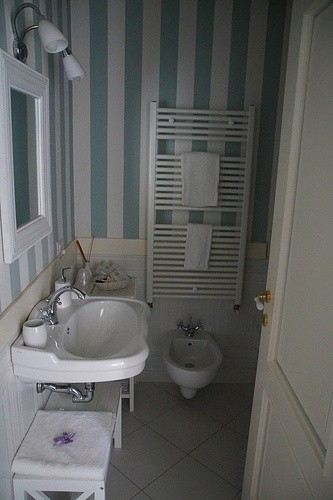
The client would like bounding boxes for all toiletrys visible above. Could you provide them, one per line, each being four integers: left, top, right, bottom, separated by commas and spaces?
54, 266, 73, 310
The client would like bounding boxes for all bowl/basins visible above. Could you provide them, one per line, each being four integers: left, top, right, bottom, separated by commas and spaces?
93, 275, 129, 290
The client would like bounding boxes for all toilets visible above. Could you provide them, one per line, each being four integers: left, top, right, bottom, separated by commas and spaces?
167, 315, 223, 401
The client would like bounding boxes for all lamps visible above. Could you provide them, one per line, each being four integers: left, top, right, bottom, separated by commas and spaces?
12, 2, 86, 82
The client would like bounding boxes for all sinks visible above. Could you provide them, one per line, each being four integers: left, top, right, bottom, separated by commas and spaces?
55, 297, 145, 369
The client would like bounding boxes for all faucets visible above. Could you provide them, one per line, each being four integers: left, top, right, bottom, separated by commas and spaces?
37, 284, 88, 327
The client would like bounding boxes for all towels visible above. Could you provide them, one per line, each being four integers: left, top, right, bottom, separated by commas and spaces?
11, 405, 117, 480
184, 224, 211, 272
181, 152, 220, 209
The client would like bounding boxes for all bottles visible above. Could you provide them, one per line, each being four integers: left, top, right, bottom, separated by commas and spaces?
75, 268, 94, 295
55, 267, 71, 308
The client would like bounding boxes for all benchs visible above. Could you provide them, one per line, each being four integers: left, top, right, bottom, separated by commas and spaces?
13, 382, 122, 500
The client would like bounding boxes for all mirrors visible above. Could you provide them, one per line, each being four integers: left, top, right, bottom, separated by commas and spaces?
0, 48, 53, 264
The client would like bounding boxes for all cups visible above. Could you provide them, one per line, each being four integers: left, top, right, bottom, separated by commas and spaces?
23, 319, 47, 349
